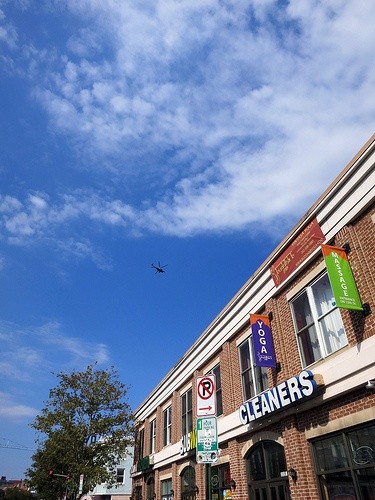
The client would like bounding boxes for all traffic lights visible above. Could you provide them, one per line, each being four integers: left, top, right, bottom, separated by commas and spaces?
48, 469, 53, 477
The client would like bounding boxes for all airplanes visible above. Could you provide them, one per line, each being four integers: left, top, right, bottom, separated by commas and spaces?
151, 260, 169, 275
